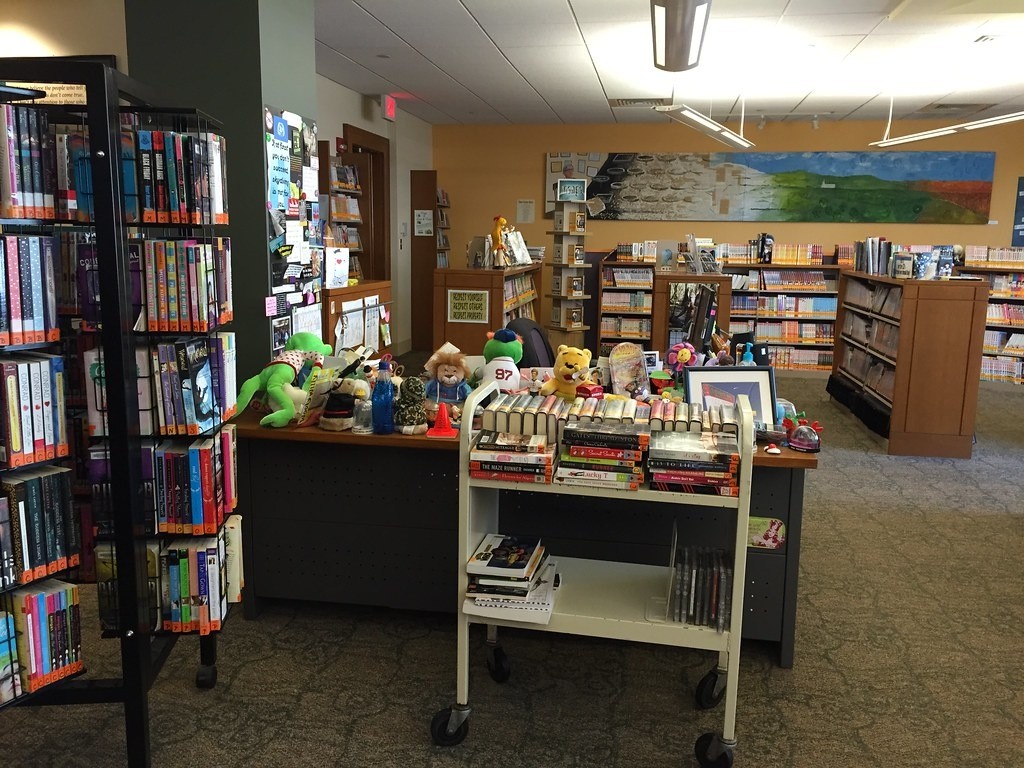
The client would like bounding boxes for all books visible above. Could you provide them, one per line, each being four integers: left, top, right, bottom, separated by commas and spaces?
470, 392, 740, 498
961, 245, 1024, 384
0, 103, 244, 703
718, 245, 855, 370
600, 240, 656, 359
466, 534, 556, 611
890, 244, 932, 257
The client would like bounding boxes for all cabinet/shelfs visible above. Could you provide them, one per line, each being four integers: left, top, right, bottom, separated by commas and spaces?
0, 54, 1024, 768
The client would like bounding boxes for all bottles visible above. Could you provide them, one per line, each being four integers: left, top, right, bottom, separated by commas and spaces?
364, 365, 376, 389
372, 363, 395, 435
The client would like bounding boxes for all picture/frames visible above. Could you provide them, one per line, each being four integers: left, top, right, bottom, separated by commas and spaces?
683, 365, 778, 430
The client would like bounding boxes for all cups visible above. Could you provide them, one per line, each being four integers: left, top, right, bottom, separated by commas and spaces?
351, 400, 372, 435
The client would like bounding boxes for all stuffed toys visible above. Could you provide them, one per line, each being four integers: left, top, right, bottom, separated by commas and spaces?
395, 329, 524, 436
229, 331, 332, 428
662, 343, 698, 403
542, 345, 592, 402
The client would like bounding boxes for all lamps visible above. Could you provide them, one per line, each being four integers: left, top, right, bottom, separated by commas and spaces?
811, 114, 820, 130
867, 94, 1024, 148
643, 80, 756, 150
649, 0, 714, 73
757, 115, 767, 130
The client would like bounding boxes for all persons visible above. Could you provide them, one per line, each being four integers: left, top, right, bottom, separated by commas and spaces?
528, 369, 543, 397
0, 660, 22, 703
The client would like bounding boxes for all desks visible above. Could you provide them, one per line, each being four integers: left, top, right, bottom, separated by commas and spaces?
232, 408, 822, 669
